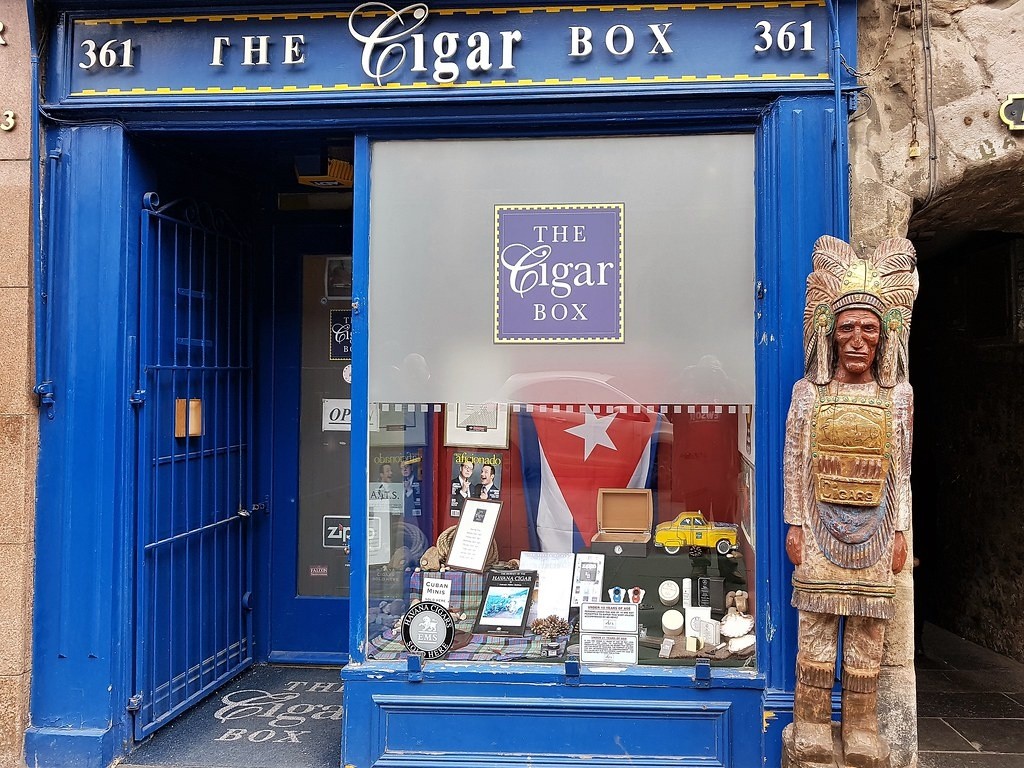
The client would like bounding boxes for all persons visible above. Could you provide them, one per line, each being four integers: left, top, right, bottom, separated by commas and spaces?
784, 235, 917, 765
374, 457, 419, 511
673, 356, 745, 583
450, 459, 499, 511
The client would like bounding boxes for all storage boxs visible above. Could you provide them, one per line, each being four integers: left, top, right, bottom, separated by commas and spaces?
590, 487, 654, 558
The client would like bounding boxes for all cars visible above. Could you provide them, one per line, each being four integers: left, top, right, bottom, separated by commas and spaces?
461, 371, 675, 490
653, 510, 739, 556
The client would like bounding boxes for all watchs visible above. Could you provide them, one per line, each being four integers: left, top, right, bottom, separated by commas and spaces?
633, 587, 640, 603
613, 586, 621, 602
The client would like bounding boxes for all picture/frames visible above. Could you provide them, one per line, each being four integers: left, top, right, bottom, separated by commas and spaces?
444, 498, 504, 573
443, 403, 510, 449
